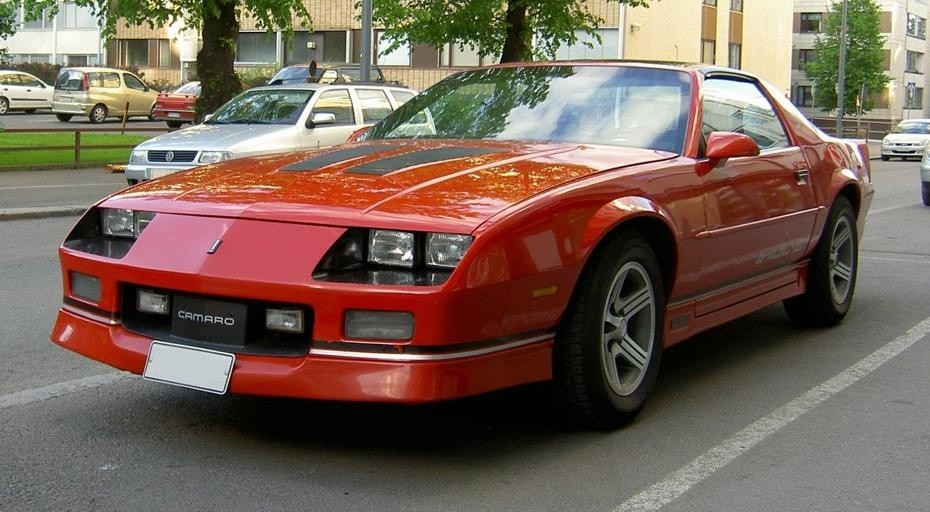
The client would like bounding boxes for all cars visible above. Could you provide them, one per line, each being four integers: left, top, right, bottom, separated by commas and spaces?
0, 70, 55, 116
879, 117, 930, 163
919, 140, 930, 207
44, 54, 873, 434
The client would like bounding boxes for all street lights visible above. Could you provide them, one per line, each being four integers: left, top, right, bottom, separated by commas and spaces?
905, 82, 917, 119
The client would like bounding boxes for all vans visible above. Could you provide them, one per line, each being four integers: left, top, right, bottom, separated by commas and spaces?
124, 81, 440, 189
